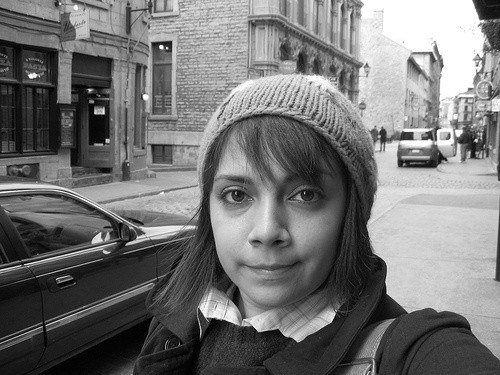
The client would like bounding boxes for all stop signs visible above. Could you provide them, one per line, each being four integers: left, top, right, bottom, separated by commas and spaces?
359, 102, 366, 110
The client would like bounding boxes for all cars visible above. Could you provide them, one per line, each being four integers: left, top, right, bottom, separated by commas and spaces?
0, 175, 197, 375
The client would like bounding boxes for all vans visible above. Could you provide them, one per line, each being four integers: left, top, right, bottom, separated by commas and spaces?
397, 127, 463, 168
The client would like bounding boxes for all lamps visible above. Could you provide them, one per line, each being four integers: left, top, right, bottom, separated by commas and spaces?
54, 1, 78, 10
352, 63, 370, 77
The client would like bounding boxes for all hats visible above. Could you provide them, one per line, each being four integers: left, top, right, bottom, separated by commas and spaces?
196, 74, 377, 226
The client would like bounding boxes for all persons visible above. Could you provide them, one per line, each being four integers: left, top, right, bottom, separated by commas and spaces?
458, 124, 489, 163
131, 74, 500, 375
371, 126, 378, 144
378, 126, 386, 152
